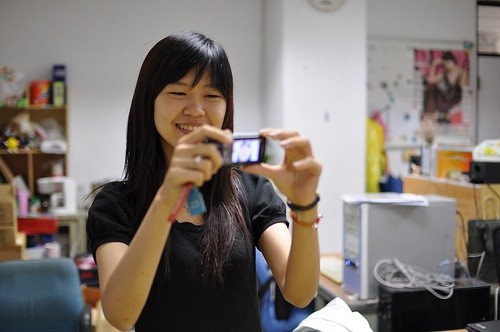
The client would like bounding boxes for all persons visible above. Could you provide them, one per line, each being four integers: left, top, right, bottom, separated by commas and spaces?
85, 31, 321, 332
235, 142, 251, 162
420, 51, 468, 120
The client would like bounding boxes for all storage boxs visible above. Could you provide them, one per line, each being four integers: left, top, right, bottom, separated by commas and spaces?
0, 184, 26, 261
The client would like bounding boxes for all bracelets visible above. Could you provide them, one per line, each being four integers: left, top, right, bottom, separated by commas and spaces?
289, 210, 323, 229
287, 194, 320, 211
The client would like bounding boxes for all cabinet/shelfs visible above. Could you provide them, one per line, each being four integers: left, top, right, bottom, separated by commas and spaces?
0, 103, 69, 199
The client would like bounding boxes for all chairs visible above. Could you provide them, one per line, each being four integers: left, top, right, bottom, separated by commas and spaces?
0, 258, 91, 332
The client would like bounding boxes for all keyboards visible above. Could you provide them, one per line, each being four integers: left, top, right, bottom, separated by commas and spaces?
320, 256, 344, 284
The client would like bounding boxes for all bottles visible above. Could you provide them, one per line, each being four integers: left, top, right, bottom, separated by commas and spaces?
51, 65, 68, 105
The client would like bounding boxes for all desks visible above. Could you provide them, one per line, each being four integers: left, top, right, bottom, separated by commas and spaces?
403, 174, 500, 268
314, 250, 380, 313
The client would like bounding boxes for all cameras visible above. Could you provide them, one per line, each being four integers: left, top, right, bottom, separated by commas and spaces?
222, 134, 267, 167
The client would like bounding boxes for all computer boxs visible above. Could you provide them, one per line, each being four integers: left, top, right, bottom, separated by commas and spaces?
342, 194, 456, 301
377, 278, 491, 332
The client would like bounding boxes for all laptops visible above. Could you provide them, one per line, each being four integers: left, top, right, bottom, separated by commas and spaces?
466, 321, 500, 332
